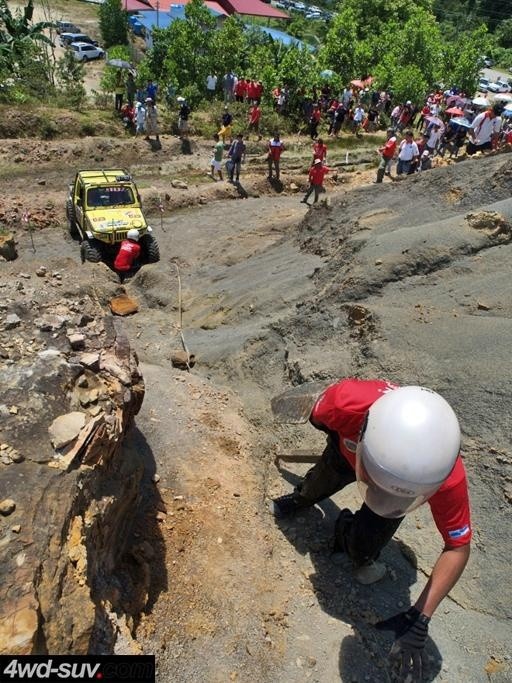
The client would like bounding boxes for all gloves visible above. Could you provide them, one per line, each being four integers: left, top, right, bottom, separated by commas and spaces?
373, 606, 436, 683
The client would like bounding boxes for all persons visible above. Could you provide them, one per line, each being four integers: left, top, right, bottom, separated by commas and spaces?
113, 229, 141, 284
267, 378, 472, 683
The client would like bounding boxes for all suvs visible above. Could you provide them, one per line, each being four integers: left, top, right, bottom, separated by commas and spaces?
67, 167, 159, 265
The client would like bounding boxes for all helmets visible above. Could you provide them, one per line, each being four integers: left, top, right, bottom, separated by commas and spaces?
355, 381, 461, 519
125, 228, 140, 242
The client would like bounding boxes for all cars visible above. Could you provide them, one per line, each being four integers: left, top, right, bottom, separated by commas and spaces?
476, 76, 490, 92
276, 0, 342, 25
57, 19, 106, 63
475, 54, 492, 68
499, 75, 512, 88
489, 80, 511, 94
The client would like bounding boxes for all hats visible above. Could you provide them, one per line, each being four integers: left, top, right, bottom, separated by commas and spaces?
176, 95, 186, 103
311, 157, 325, 167
135, 96, 154, 109
493, 104, 504, 116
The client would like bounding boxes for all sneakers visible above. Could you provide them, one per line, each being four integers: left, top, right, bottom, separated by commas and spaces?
297, 198, 309, 204
330, 509, 356, 567
205, 172, 240, 185
264, 175, 281, 180
266, 486, 315, 520
